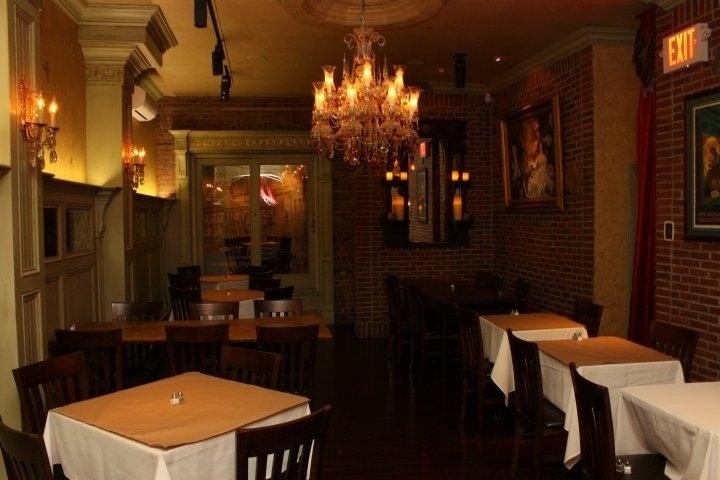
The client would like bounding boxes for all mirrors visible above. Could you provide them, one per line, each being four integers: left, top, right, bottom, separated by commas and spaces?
397, 119, 471, 246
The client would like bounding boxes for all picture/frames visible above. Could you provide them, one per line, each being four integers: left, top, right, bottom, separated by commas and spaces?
500, 92, 565, 213
417, 167, 428, 223
682, 87, 720, 240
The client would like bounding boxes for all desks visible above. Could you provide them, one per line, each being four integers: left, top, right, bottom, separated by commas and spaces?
615, 380, 720, 480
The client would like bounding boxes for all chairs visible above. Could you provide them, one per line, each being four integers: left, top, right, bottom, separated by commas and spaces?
480, 269, 533, 308
220, 234, 293, 274
379, 271, 456, 376
454, 305, 504, 436
574, 296, 604, 336
505, 328, 565, 479
568, 361, 651, 480
650, 319, 699, 379
0, 263, 338, 480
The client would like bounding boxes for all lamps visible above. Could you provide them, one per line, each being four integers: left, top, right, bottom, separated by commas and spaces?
449, 171, 471, 221
192, 0, 235, 101
386, 171, 408, 221
123, 142, 148, 186
19, 90, 60, 167
661, 22, 711, 75
306, 0, 423, 167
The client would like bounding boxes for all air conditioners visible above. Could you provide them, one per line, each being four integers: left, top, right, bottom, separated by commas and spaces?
132, 85, 159, 123
